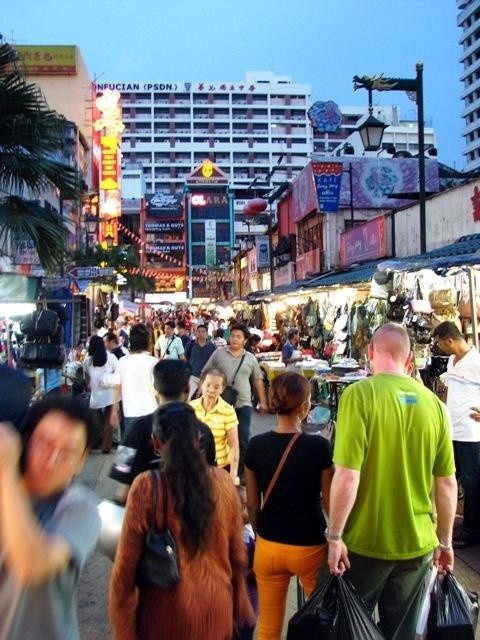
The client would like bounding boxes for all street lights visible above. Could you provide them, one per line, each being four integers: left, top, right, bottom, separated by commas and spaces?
243, 213, 274, 291
82, 210, 98, 261
105, 234, 114, 258
352, 62, 427, 257
222, 244, 244, 298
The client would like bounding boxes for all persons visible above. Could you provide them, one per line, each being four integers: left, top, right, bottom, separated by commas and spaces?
1, 302, 480, 640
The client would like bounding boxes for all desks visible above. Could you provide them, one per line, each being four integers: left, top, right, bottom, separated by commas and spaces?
252, 346, 369, 422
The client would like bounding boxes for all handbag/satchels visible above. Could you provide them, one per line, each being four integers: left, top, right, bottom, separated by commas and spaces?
132, 522, 183, 594
100, 369, 123, 390
219, 384, 239, 408
